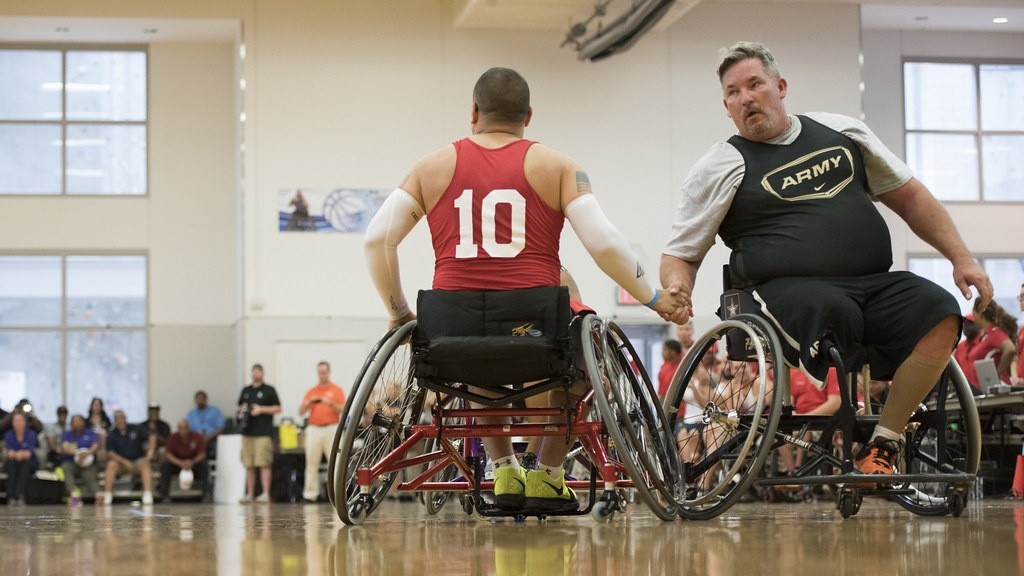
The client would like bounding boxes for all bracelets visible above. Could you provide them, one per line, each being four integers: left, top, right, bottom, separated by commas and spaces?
644, 291, 659, 307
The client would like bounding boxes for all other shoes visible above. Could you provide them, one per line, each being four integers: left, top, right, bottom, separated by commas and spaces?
69, 498, 85, 507
104, 492, 113, 504
239, 495, 255, 503
256, 493, 271, 502
141, 490, 154, 503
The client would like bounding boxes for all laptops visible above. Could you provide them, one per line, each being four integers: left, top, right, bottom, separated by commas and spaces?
974, 358, 1001, 394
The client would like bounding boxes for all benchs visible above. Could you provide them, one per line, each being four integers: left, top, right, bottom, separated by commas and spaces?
0, 460, 215, 502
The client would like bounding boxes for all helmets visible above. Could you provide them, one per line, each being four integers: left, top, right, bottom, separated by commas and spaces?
148, 403, 160, 411
73, 447, 94, 468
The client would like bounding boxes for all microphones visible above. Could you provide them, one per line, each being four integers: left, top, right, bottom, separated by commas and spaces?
989, 384, 1024, 393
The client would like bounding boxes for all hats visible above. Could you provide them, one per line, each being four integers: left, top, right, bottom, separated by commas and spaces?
963, 314, 974, 323
179, 468, 194, 490
666, 341, 682, 354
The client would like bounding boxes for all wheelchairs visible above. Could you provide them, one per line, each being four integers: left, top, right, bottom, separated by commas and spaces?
327, 285, 685, 526
662, 264, 983, 520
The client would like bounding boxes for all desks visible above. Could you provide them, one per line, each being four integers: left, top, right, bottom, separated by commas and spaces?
921, 389, 1024, 490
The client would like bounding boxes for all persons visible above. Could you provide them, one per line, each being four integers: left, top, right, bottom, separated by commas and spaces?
366, 67, 693, 508
0, 286, 1024, 505
658, 42, 992, 490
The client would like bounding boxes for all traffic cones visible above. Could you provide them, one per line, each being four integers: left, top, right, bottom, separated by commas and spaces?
1012, 455, 1024, 497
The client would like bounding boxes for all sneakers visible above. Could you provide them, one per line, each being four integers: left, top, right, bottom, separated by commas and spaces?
852, 435, 900, 487
523, 469, 579, 508
493, 467, 527, 508
